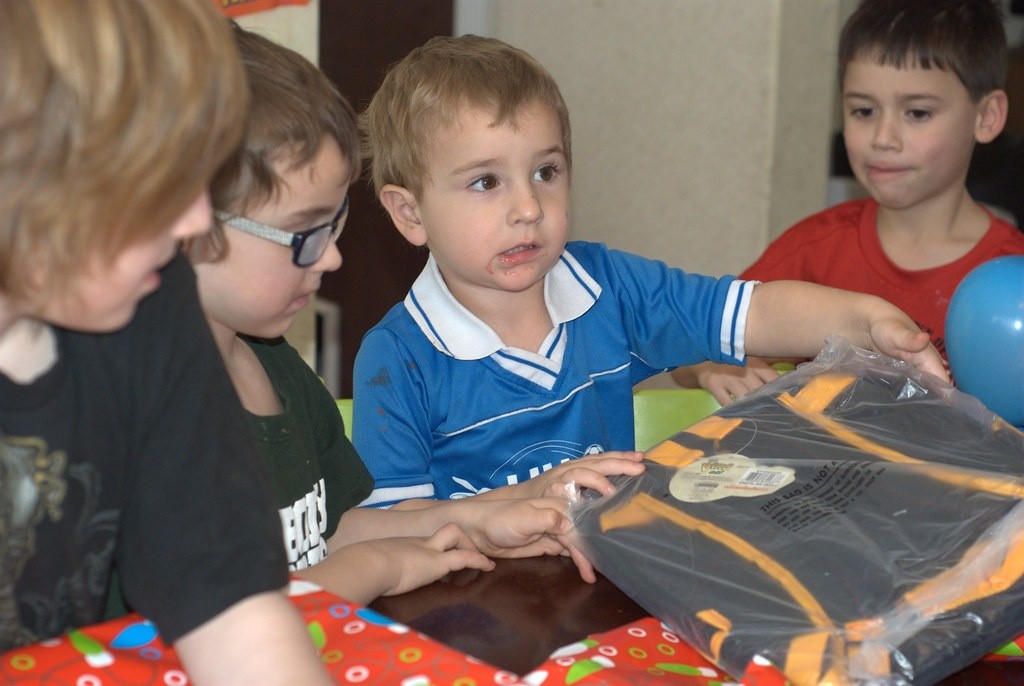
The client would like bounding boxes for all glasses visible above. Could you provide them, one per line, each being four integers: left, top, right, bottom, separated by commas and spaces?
214, 197, 350, 269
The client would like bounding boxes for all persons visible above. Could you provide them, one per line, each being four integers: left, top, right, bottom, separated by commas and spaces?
0, 0, 333, 686
353, 34, 958, 515
162, 24, 649, 615
664, 2, 1024, 414
